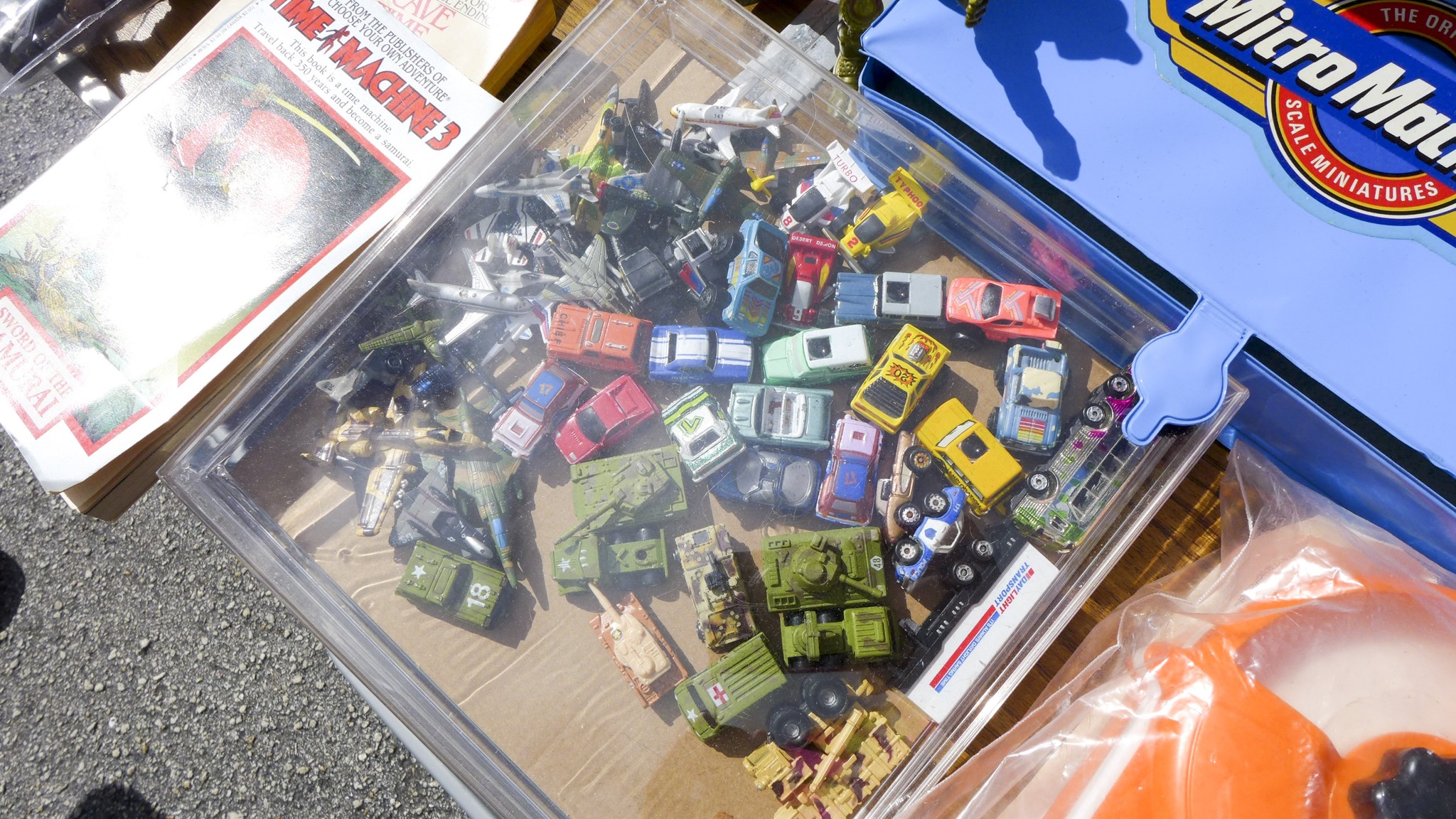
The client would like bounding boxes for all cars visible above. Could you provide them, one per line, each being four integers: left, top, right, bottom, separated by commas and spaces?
299, 71, 1141, 819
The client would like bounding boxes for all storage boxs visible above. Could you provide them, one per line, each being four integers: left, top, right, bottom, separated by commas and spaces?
150, 0, 1252, 818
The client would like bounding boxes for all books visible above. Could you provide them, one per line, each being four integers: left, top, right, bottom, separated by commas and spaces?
1, 0, 503, 517
